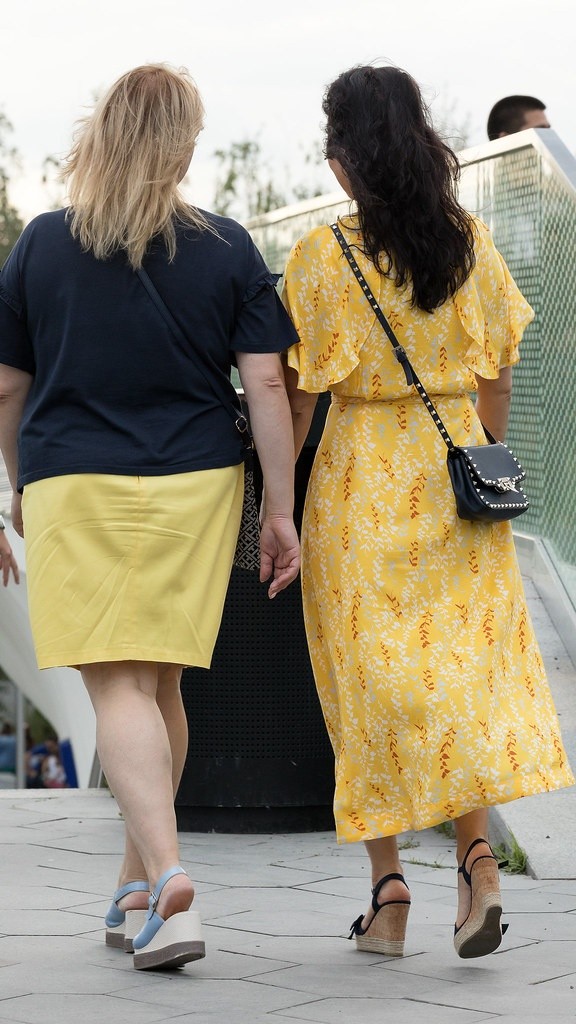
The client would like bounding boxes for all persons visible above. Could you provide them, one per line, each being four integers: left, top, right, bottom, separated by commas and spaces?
0, 65, 301, 969
0, 529, 20, 587
0, 719, 67, 789
477, 95, 551, 261
259, 65, 576, 959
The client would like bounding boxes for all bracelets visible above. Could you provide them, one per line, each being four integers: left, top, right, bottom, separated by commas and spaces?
0, 515, 5, 529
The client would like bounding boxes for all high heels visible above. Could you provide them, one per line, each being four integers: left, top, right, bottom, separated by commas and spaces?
454, 838, 509, 959
105, 881, 149, 953
133, 866, 206, 969
347, 873, 411, 957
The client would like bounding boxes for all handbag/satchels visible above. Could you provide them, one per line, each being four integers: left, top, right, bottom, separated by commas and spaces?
447, 443, 530, 522
233, 437, 263, 572
